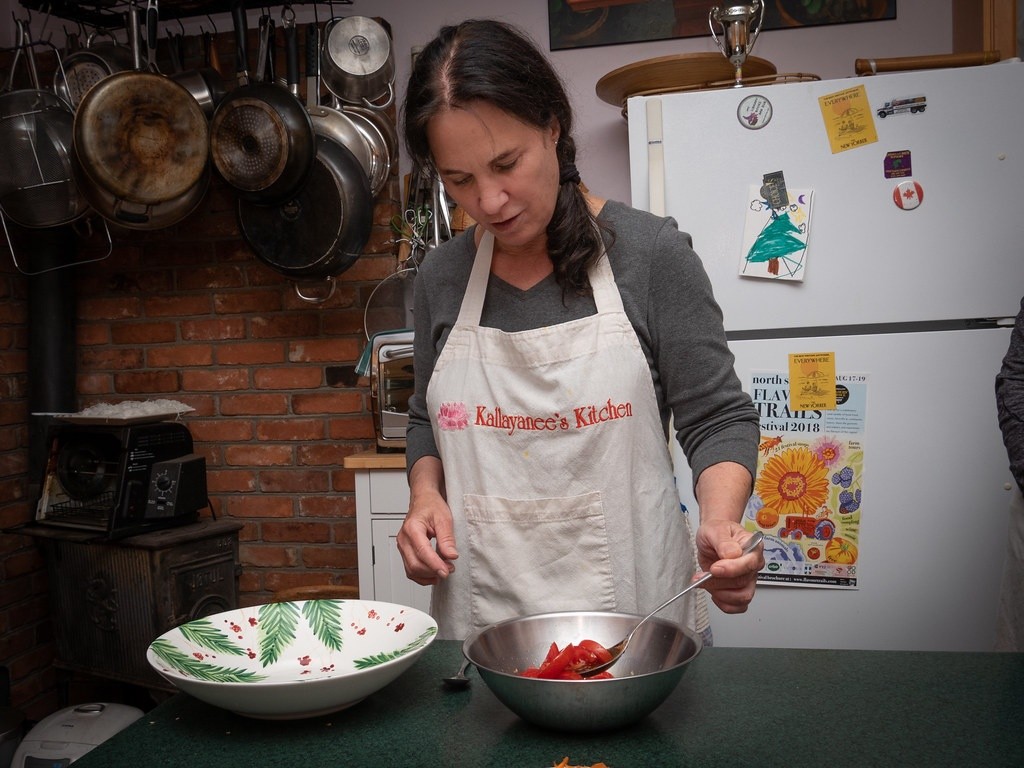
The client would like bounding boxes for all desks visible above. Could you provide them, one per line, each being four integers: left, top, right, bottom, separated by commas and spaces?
61, 638, 1024, 768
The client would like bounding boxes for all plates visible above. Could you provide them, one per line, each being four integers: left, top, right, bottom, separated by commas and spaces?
145, 596, 440, 722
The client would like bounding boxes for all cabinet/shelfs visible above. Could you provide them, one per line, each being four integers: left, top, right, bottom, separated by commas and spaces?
345, 450, 438, 616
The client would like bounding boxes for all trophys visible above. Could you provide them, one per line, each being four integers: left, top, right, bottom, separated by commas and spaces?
708, 0, 764, 89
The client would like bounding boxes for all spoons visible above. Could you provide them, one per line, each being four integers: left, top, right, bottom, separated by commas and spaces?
441, 655, 472, 688
573, 530, 765, 678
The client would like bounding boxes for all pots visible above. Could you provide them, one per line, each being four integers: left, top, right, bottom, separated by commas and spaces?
234, 0, 399, 305
70, 0, 215, 232
207, 14, 316, 207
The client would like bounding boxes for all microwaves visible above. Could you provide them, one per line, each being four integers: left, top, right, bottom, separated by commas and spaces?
369, 330, 416, 455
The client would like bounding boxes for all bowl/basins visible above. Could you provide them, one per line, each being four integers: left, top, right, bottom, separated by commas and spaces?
461, 610, 704, 734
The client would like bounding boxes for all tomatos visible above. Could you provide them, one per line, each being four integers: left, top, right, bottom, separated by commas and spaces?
513, 640, 615, 679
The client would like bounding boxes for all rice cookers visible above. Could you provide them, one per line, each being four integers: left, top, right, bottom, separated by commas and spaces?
8, 702, 146, 768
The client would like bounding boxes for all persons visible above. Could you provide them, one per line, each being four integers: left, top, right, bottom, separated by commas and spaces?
995, 298, 1024, 654
396, 20, 764, 649
